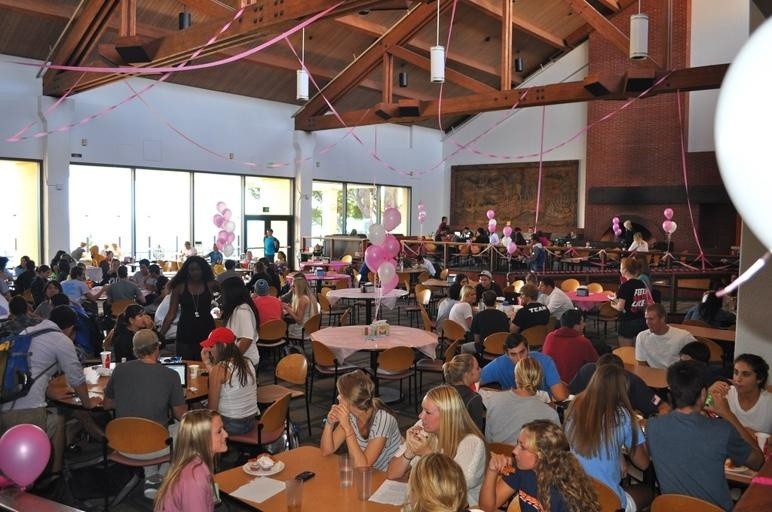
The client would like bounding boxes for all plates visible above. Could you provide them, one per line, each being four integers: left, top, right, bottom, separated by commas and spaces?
242, 461, 286, 477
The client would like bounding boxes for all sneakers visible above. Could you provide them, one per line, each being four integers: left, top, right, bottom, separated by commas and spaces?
110, 471, 140, 508
144, 478, 165, 501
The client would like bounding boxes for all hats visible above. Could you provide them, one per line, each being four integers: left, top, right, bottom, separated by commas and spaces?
477, 270, 493, 279
199, 327, 237, 349
254, 279, 271, 296
133, 329, 162, 351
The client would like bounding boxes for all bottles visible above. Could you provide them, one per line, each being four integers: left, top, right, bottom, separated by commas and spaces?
364, 320, 390, 336
121, 357, 127, 364
51, 271, 57, 282
86, 278, 92, 289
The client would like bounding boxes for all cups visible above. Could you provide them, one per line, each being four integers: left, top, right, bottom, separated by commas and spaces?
337, 453, 372, 501
189, 364, 201, 379
478, 298, 525, 313
754, 432, 770, 456
100, 351, 111, 370
283, 478, 304, 512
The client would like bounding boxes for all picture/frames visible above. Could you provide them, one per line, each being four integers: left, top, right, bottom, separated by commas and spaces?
450, 160, 580, 233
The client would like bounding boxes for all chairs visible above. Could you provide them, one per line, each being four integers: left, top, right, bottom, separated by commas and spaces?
586, 479, 624, 512
405, 233, 671, 271
486, 443, 516, 464
257, 354, 312, 438
650, 494, 723, 512
406, 269, 460, 331
226, 392, 292, 459
310, 325, 464, 411
103, 416, 175, 512
441, 278, 620, 364
257, 312, 322, 365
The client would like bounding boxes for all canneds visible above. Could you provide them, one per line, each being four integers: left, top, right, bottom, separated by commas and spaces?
704, 393, 713, 406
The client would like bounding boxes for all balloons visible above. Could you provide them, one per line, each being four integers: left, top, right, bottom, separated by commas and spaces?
216, 201, 226, 216
223, 209, 231, 221
417, 202, 427, 226
382, 207, 401, 233
664, 208, 674, 220
486, 209, 495, 220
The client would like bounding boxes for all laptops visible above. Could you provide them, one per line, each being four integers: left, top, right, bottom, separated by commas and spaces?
163, 363, 188, 398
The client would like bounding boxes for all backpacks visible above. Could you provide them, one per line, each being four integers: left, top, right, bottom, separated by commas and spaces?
0, 326, 64, 404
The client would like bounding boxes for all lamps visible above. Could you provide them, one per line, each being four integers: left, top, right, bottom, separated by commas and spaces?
515, 49, 524, 73
178, 4, 192, 32
398, 60, 410, 89
428, 0, 446, 85
296, 26, 310, 104
628, 0, 650, 62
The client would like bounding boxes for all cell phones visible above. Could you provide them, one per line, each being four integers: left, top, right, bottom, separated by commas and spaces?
295, 470, 315, 482
